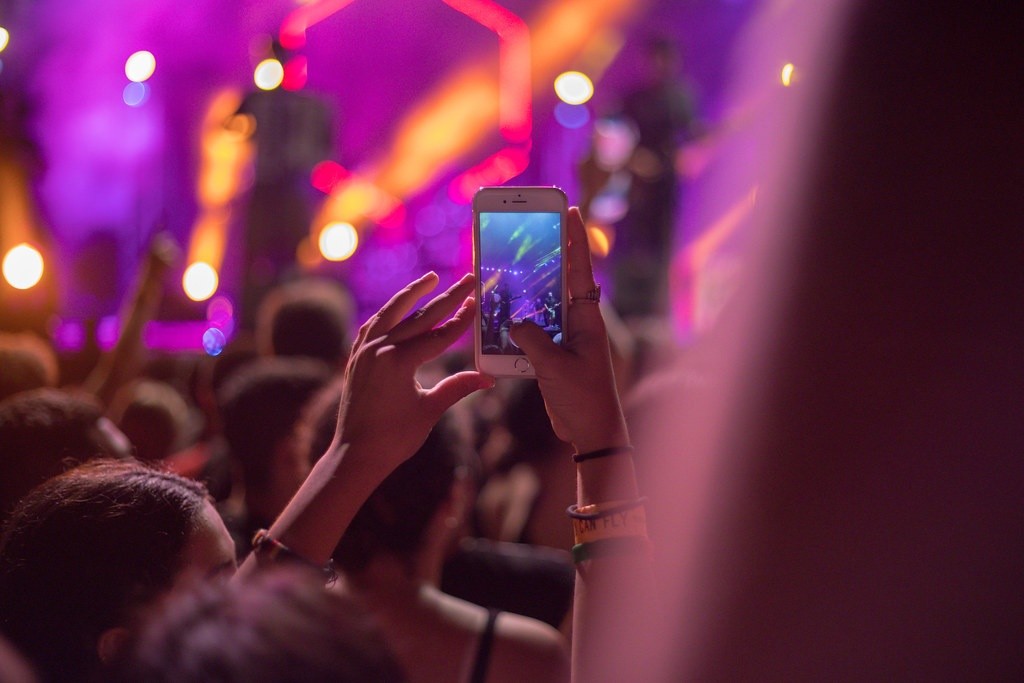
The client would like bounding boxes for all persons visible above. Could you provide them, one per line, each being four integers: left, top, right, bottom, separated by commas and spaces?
0, 37, 687, 683
543, 291, 559, 328
494, 283, 512, 325
484, 293, 501, 345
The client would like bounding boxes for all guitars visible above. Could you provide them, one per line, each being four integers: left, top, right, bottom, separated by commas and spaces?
546, 302, 561, 313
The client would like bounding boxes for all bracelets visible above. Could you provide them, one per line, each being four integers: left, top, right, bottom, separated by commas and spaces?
566, 495, 649, 522
573, 499, 651, 543
571, 536, 651, 563
251, 530, 340, 592
572, 445, 636, 462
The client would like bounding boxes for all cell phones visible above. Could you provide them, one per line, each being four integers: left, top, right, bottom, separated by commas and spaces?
472, 186, 569, 379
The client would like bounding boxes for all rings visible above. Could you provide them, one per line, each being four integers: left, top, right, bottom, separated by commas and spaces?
570, 283, 602, 302
